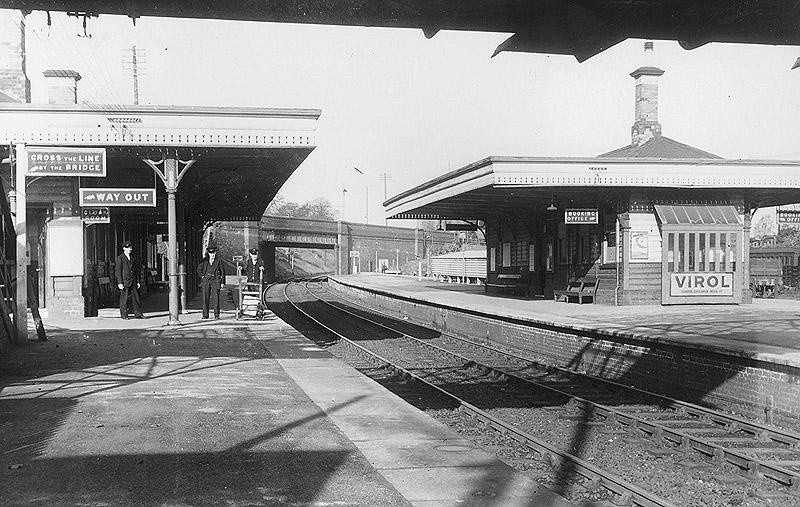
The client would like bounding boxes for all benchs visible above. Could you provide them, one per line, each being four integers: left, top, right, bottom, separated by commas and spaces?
150, 272, 169, 294
553, 276, 600, 305
93, 275, 120, 309
485, 274, 522, 296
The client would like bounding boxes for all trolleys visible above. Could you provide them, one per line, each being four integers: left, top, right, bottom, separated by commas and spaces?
234, 266, 266, 320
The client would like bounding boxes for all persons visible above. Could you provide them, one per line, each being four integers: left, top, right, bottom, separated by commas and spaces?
238, 247, 266, 315
114, 240, 147, 319
197, 246, 225, 318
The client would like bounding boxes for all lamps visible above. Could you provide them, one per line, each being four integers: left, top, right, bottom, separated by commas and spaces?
436, 216, 444, 231
547, 192, 557, 211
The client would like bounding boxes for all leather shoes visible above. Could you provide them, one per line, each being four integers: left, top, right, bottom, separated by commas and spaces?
123, 313, 146, 320
202, 317, 222, 320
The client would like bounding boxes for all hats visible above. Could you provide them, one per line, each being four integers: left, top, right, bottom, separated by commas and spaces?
249, 248, 258, 254
206, 247, 217, 252
121, 240, 133, 247
